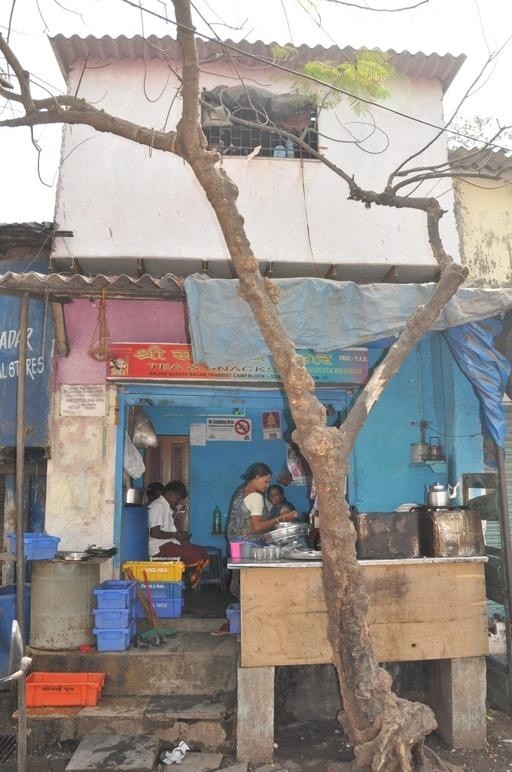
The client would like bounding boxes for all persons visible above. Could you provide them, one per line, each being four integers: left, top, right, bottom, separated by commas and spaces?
218, 462, 298, 637
267, 484, 295, 517
146, 479, 210, 569
144, 481, 165, 505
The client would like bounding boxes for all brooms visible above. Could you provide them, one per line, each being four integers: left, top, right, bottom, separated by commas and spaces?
123, 565, 165, 648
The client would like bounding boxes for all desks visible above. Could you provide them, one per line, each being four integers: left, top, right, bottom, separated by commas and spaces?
226, 554, 490, 669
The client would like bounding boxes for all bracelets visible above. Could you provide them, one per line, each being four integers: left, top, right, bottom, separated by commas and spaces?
277, 517, 279, 523
282, 514, 285, 521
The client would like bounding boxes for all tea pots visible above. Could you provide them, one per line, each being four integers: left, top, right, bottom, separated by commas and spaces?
426, 482, 459, 511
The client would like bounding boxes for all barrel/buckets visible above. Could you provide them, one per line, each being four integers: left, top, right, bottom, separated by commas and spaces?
429, 435, 442, 462
0, 582, 31, 686
412, 441, 427, 463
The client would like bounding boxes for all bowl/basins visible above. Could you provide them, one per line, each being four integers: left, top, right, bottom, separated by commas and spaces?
122, 489, 146, 505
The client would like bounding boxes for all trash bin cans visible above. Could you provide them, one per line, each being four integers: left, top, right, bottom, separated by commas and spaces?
226, 603, 240, 634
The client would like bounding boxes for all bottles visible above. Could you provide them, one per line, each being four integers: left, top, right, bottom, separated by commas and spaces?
411, 442, 443, 465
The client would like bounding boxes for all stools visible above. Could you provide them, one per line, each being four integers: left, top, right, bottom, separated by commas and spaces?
198, 545, 226, 592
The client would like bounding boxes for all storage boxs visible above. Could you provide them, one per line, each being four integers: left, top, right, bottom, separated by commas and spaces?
6, 531, 186, 708
